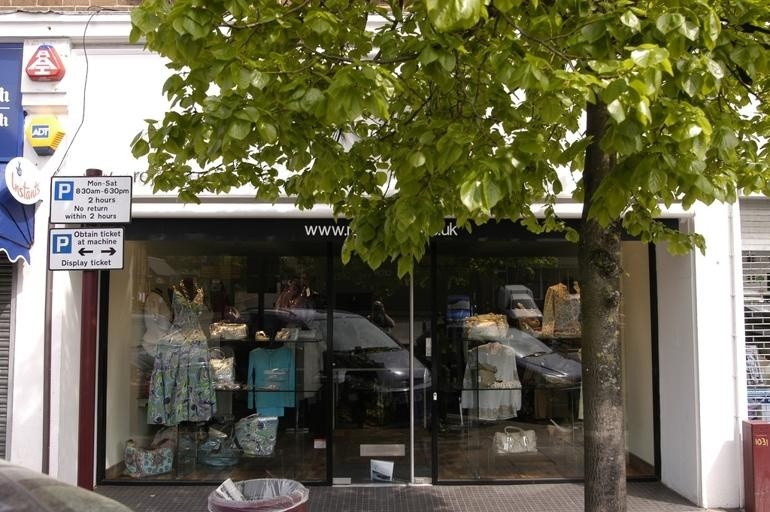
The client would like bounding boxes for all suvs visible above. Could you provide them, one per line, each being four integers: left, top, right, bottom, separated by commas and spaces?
245, 307, 431, 424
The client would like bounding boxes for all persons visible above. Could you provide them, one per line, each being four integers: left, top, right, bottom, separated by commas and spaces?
142, 288, 172, 359
339, 371, 366, 427
147, 276, 218, 426
544, 278, 582, 338
204, 278, 229, 320
360, 301, 394, 334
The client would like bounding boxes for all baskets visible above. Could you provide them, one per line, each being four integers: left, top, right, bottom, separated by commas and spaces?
301, 329, 316, 338
275, 327, 300, 339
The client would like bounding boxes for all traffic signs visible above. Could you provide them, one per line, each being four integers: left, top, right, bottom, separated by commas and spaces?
50, 175, 133, 224
49, 230, 125, 271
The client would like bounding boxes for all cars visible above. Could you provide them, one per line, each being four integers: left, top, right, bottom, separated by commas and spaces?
446, 293, 476, 330
416, 326, 582, 417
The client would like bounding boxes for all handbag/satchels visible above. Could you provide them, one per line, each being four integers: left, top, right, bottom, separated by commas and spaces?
208, 347, 235, 383
234, 413, 277, 455
469, 312, 510, 339
208, 426, 234, 451
124, 436, 175, 478
493, 424, 536, 454
210, 319, 248, 341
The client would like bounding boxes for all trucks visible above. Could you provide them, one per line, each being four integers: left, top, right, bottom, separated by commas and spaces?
497, 283, 542, 326
